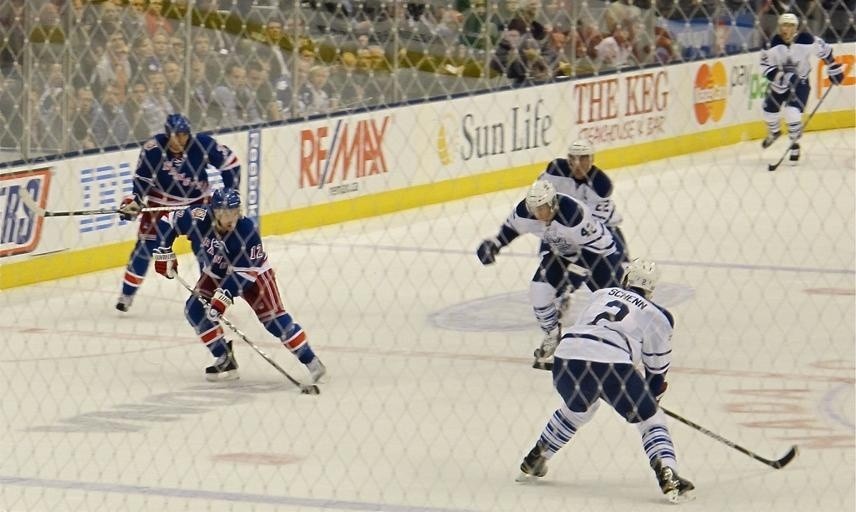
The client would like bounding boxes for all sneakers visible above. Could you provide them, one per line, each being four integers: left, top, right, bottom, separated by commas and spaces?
788, 141, 800, 161
760, 131, 780, 147
115, 292, 134, 310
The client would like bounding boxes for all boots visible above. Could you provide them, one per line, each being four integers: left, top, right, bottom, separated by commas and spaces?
518, 439, 549, 476
204, 340, 239, 372
533, 323, 562, 359
654, 459, 695, 495
307, 354, 326, 379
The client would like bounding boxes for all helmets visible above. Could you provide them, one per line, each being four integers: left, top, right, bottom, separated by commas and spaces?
620, 256, 657, 293
164, 113, 191, 136
524, 180, 557, 216
212, 188, 240, 209
567, 139, 595, 179
777, 13, 799, 29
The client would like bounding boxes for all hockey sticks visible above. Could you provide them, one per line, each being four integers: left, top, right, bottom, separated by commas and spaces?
17, 187, 191, 218
169, 268, 320, 395
663, 403, 799, 469
767, 85, 834, 170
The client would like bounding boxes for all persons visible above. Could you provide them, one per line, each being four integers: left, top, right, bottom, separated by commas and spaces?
116, 113, 240, 312
154, 188, 327, 384
520, 257, 695, 495
536, 141, 629, 318
477, 179, 622, 359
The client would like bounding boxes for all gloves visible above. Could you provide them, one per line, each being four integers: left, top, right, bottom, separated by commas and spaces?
150, 246, 178, 280
475, 237, 502, 264
771, 70, 799, 87
826, 61, 843, 86
202, 288, 234, 321
120, 191, 144, 222
552, 263, 591, 298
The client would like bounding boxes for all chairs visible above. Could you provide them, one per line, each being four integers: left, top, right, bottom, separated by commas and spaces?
0, 0, 761, 155
